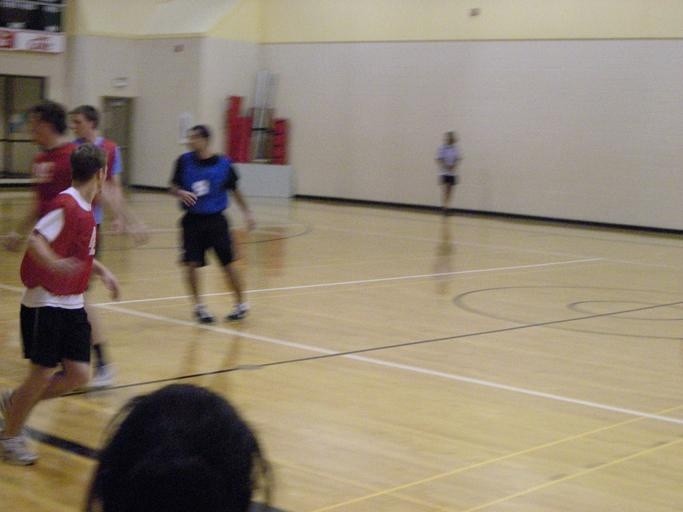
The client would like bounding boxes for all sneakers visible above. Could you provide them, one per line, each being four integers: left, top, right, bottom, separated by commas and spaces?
89, 359, 114, 389
226, 301, 252, 320
1, 429, 40, 467
193, 302, 215, 320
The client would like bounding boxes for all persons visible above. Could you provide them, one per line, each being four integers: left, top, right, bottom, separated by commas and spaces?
432, 129, 465, 217
1, 141, 122, 469
166, 123, 259, 325
63, 103, 125, 261
81, 382, 273, 512
0, 97, 150, 394
